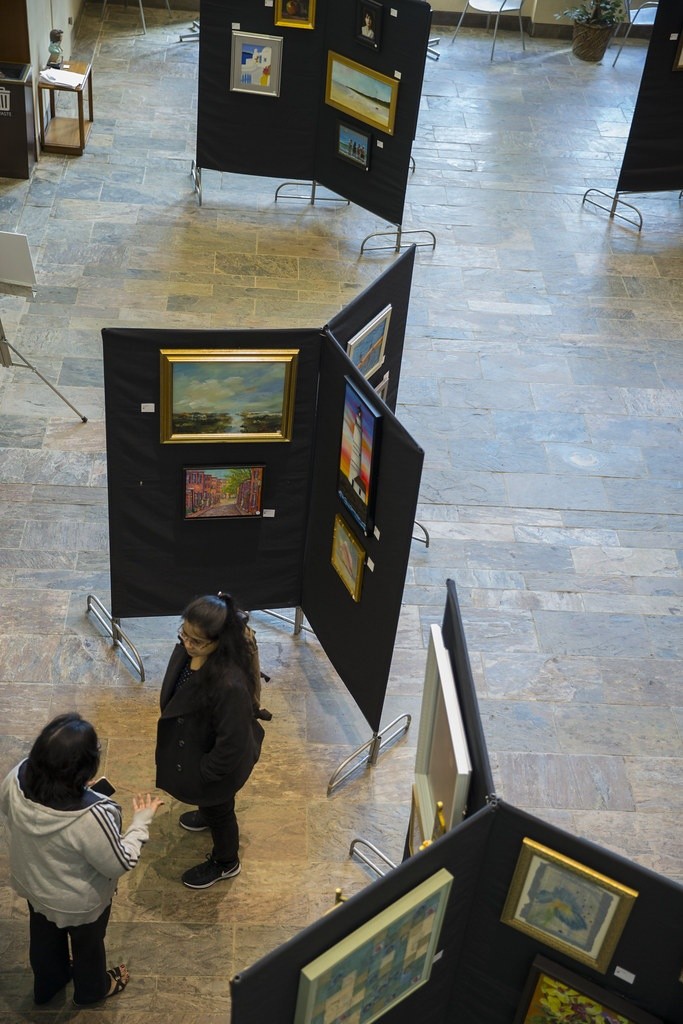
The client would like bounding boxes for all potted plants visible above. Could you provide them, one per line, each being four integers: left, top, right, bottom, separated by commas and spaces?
555, 0, 626, 61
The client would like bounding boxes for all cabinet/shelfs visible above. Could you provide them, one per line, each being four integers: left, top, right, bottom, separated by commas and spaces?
38, 61, 91, 156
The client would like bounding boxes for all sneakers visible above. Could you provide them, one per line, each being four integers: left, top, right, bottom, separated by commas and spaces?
178, 809, 210, 831
182, 854, 240, 888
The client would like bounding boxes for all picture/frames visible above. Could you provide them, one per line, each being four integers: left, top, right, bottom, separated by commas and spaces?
500, 836, 638, 974
331, 376, 383, 536
515, 953, 683, 1024
295, 866, 453, 1024
354, 0, 384, 51
347, 304, 393, 380
410, 624, 473, 859
274, 0, 317, 30
230, 30, 284, 97
333, 119, 371, 172
331, 514, 366, 602
180, 465, 264, 521
159, 350, 299, 445
374, 378, 389, 402
326, 49, 400, 137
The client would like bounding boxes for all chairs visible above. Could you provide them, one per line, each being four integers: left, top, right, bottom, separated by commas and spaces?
608, 1, 659, 66
453, 0, 526, 61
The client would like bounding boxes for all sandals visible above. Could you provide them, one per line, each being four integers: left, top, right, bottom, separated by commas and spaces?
73, 966, 129, 1006
68, 959, 74, 982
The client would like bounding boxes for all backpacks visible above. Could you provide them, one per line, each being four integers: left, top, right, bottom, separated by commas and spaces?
233, 620, 273, 722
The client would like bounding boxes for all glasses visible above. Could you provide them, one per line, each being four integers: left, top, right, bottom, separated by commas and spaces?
176, 623, 215, 650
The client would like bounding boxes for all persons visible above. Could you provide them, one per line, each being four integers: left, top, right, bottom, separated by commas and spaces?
0, 713, 165, 1006
155, 592, 266, 888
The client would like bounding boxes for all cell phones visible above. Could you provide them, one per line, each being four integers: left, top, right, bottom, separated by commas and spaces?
89, 777, 115, 798
